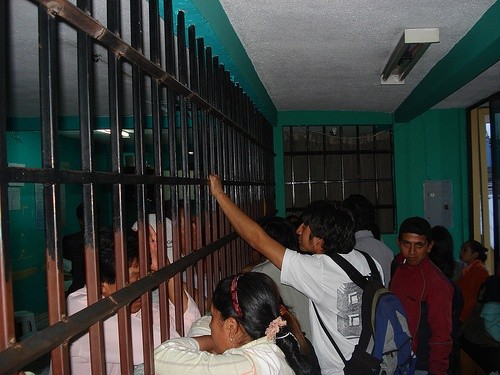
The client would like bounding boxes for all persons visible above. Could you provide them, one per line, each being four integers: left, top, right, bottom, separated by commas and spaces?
62, 171, 217, 375
241, 194, 393, 350
390, 217, 500, 375
154, 272, 321, 375
208, 172, 418, 375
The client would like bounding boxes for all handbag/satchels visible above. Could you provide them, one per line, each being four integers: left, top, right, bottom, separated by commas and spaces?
455, 284, 500, 349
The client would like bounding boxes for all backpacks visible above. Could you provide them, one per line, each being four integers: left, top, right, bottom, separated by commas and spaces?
312, 246, 418, 375
